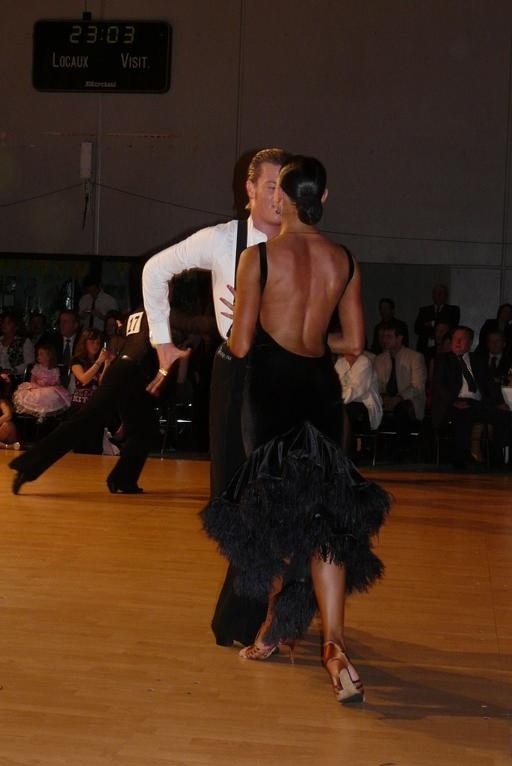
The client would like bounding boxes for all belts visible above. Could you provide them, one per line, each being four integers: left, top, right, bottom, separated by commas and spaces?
118, 353, 135, 361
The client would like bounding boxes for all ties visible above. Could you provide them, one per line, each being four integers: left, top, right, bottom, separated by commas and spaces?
63, 339, 71, 364
458, 353, 476, 393
385, 358, 398, 395
488, 356, 499, 376
89, 298, 94, 328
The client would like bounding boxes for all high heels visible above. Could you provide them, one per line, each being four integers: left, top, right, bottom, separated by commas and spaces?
238, 624, 295, 664
322, 641, 364, 703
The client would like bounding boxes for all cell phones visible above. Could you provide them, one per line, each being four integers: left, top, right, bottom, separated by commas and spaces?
103, 341, 107, 349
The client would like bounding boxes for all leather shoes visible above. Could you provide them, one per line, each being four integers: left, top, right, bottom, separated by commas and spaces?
108, 436, 126, 445
8, 458, 24, 494
6, 442, 21, 451
106, 481, 144, 494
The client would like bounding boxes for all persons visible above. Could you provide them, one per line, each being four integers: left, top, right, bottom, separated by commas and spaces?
199, 155, 397, 705
141, 149, 299, 650
1, 273, 222, 495
335, 284, 511, 472
